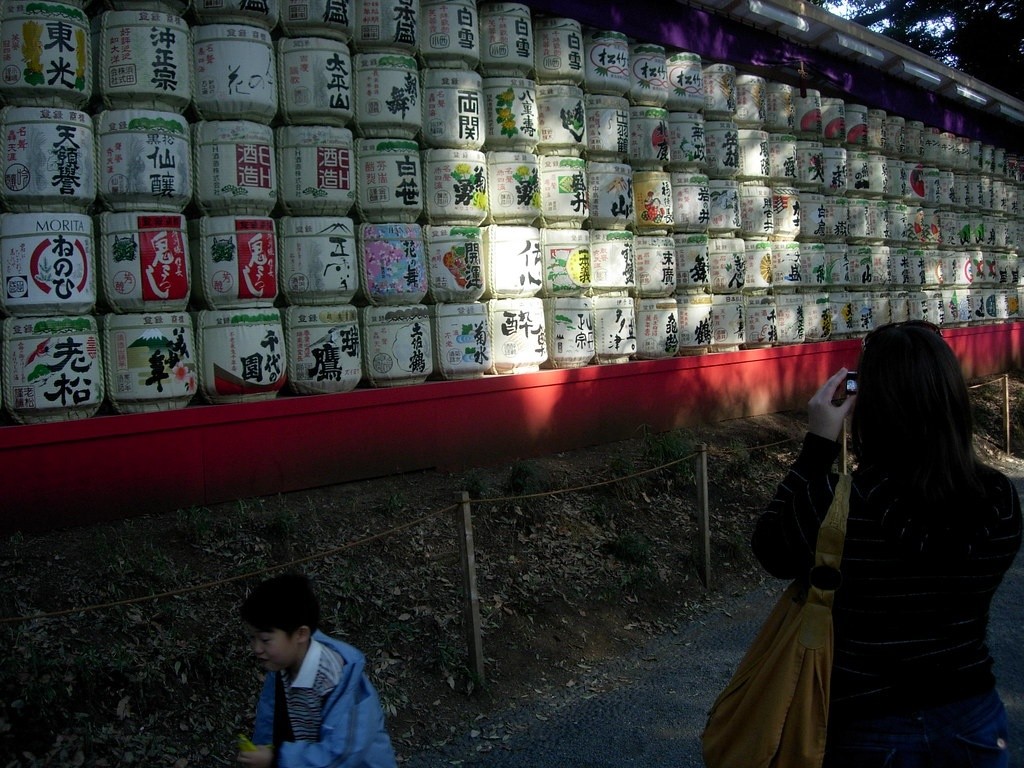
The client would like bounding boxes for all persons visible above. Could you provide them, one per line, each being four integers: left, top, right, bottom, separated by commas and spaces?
751, 320, 1023, 768
237, 573, 400, 768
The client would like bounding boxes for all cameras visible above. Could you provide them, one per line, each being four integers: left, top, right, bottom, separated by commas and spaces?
845, 372, 858, 395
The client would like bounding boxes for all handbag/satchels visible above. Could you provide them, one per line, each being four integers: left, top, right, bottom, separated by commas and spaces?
701, 472, 852, 768
